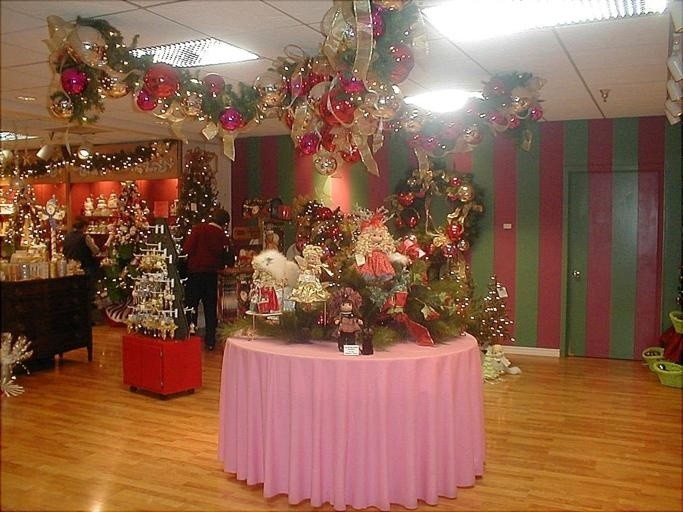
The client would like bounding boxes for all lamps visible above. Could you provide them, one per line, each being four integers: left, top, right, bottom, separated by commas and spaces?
36, 145, 53, 162
77, 141, 92, 160
665, 7, 683, 125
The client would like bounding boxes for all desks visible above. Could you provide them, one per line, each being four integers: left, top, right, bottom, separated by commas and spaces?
218, 332, 485, 511
122, 333, 202, 400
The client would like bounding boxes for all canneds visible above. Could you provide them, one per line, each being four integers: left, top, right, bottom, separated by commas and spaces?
20, 262, 30, 280
60, 260, 67, 277
50, 262, 57, 278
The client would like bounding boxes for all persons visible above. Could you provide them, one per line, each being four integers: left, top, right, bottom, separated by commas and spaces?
63, 219, 100, 328
183, 208, 235, 350
251, 218, 419, 354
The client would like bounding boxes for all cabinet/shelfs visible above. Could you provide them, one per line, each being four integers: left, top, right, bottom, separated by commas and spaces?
85, 216, 121, 258
0, 275, 92, 361
